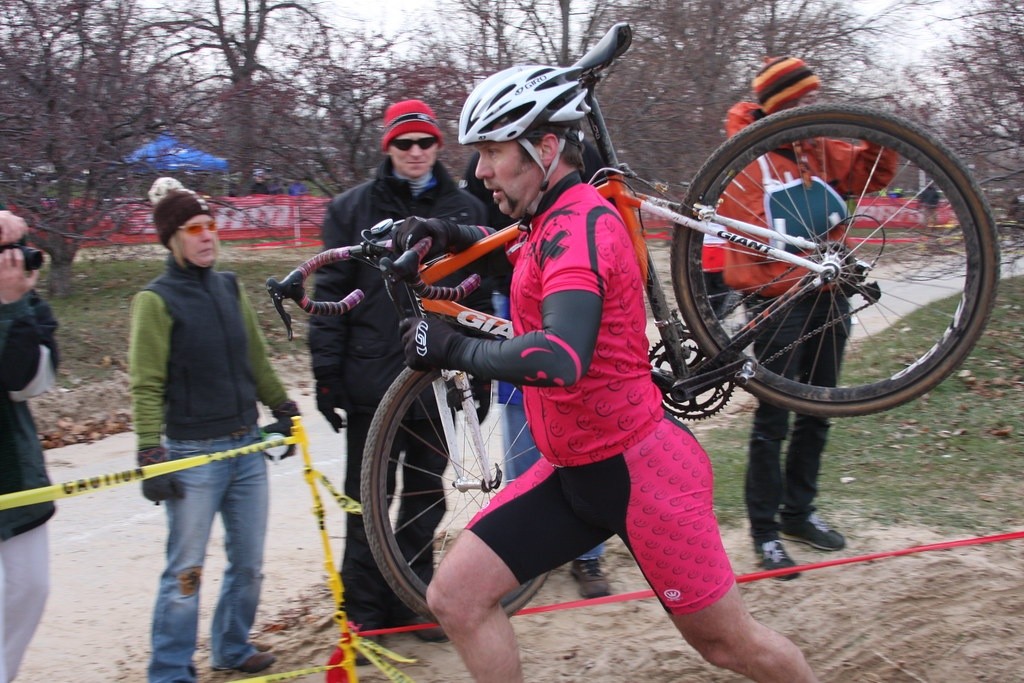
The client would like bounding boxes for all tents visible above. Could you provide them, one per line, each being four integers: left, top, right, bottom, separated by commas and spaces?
108, 134, 230, 194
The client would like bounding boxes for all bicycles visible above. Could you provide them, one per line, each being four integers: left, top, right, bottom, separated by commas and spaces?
265, 19, 1000, 629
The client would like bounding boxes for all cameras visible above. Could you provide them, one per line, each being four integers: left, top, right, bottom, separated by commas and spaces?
1, 243, 44, 271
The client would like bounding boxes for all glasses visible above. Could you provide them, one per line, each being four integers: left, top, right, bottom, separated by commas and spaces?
390, 136, 436, 151
177, 220, 217, 235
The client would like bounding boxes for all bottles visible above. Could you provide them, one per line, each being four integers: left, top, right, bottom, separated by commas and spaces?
259, 419, 289, 457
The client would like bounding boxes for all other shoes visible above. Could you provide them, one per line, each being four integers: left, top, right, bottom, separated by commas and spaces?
403, 616, 446, 643
212, 651, 276, 673
354, 632, 377, 666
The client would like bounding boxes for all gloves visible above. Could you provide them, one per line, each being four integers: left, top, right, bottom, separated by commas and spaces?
137, 447, 185, 506
399, 318, 465, 370
262, 401, 301, 460
319, 402, 344, 434
393, 215, 460, 262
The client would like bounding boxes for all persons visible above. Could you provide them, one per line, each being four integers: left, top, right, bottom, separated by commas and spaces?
391, 66, 820, 683
309, 100, 494, 667
878, 179, 940, 234
127, 176, 302, 683
461, 150, 611, 599
723, 55, 897, 582
0, 210, 62, 683
247, 168, 304, 197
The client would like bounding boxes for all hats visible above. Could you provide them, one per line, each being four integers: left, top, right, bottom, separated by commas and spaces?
381, 99, 443, 152
751, 56, 821, 114
149, 176, 215, 250
458, 65, 589, 146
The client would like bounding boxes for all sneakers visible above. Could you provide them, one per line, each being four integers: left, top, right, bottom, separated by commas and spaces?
569, 559, 611, 599
756, 540, 799, 580
778, 509, 845, 552
